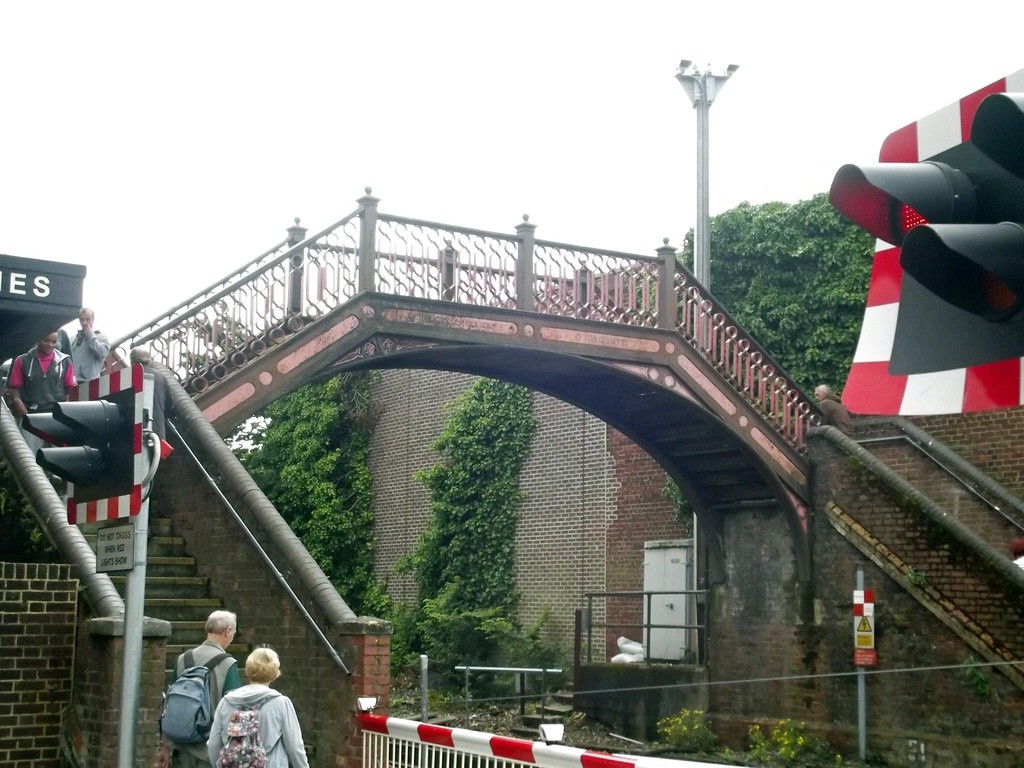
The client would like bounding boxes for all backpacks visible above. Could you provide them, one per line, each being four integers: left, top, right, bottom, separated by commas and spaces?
161, 649, 233, 744
216, 694, 282, 768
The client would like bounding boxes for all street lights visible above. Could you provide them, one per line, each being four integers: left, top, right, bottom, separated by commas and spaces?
674, 58, 746, 291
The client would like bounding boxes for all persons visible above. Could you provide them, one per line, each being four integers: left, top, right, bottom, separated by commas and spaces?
207, 648, 309, 768
814, 384, 855, 437
4, 307, 111, 498
157, 611, 242, 768
1010, 537, 1024, 571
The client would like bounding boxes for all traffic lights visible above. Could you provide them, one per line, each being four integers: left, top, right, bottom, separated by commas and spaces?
20, 360, 143, 525
825, 66, 1024, 420
